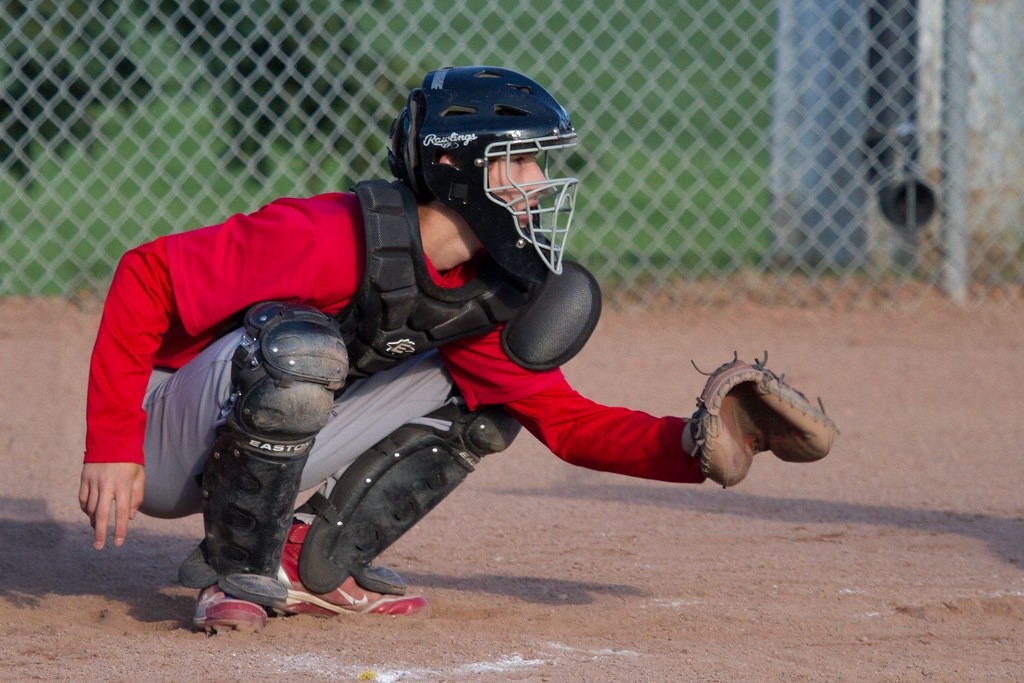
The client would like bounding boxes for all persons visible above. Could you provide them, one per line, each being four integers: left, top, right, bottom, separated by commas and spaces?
79, 65, 835, 638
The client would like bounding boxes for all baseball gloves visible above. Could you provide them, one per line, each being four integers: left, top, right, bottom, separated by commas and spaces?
693, 364, 836, 490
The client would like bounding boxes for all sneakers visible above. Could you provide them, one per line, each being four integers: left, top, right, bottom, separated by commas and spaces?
272, 524, 428, 618
193, 582, 267, 632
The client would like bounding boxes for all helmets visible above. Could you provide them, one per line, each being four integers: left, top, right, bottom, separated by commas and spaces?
387, 67, 578, 286
